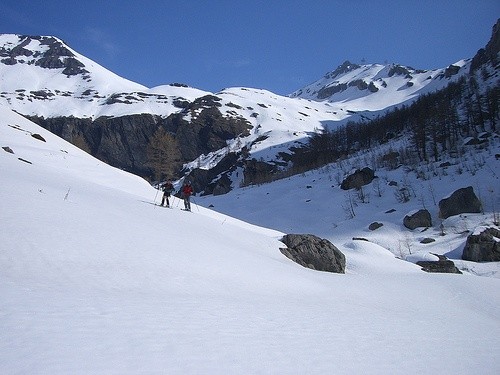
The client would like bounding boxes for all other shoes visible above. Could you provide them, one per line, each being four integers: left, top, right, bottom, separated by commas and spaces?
158, 204, 193, 211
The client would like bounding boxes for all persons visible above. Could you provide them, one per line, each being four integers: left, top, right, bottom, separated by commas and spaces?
159, 178, 175, 207
181, 180, 195, 212
